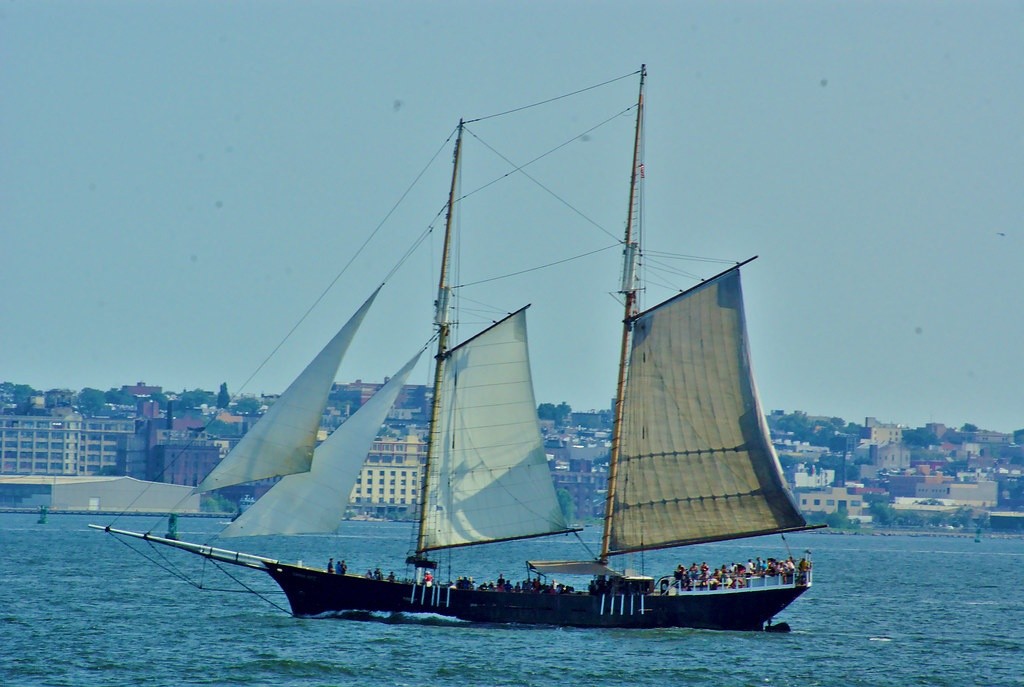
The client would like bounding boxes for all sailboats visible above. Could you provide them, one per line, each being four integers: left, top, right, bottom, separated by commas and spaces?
86, 59, 831, 626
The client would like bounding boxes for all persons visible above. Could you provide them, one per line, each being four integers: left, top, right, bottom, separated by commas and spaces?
458, 573, 575, 594
366, 567, 395, 582
588, 572, 643, 594
424, 572, 434, 587
328, 557, 347, 575
674, 555, 813, 590
